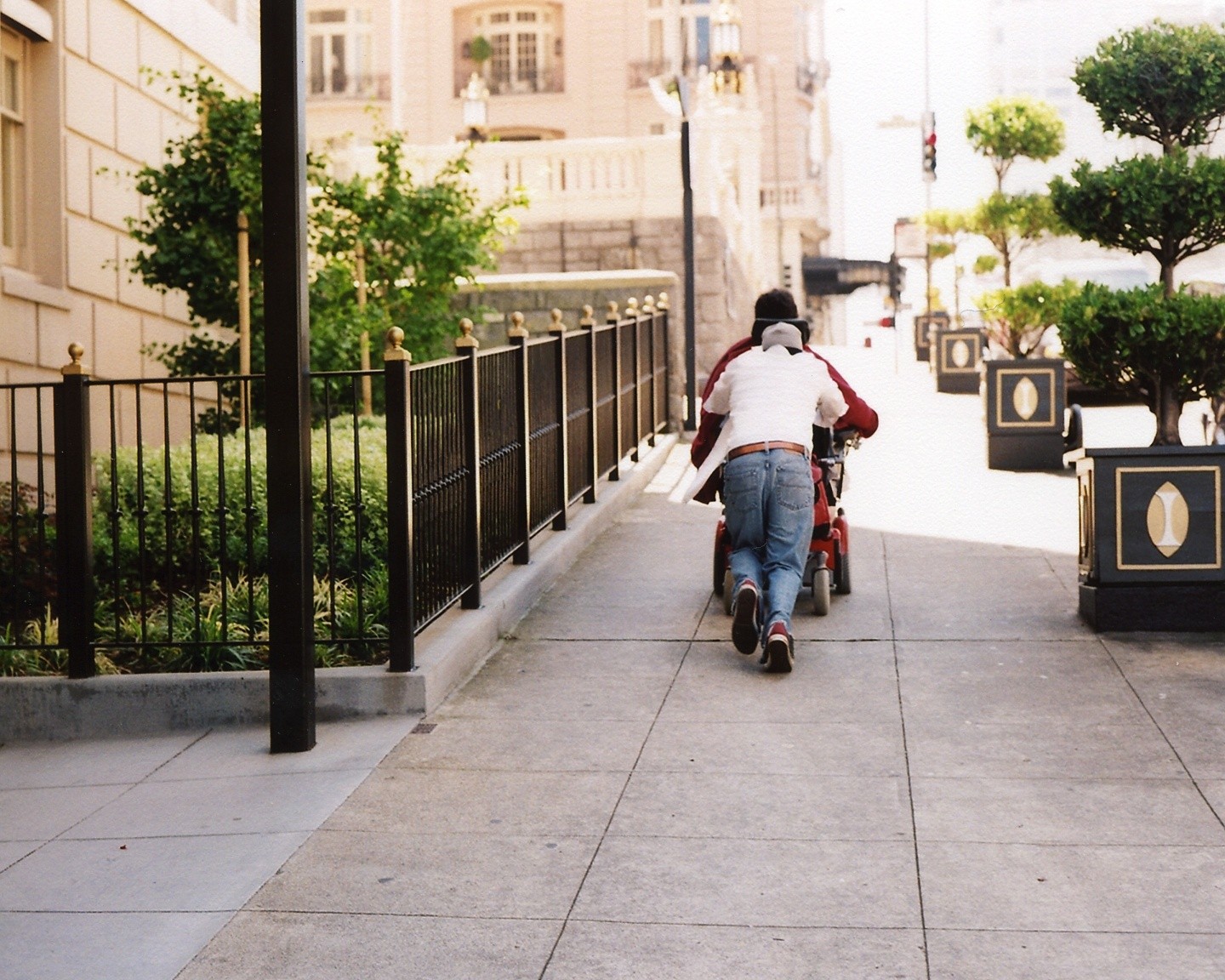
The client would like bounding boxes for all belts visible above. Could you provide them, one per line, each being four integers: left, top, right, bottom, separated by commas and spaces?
727, 441, 810, 461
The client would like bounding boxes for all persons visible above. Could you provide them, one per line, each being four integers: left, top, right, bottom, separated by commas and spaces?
702, 321, 851, 673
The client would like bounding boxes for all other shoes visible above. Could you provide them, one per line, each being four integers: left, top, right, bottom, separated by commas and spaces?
766, 622, 794, 672
732, 578, 760, 655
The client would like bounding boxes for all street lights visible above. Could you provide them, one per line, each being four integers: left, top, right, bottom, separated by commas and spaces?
649, 54, 743, 433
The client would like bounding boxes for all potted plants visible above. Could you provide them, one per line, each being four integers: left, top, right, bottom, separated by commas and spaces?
957, 100, 1065, 472
1056, 16, 1225, 639
923, 204, 983, 397
915, 209, 966, 361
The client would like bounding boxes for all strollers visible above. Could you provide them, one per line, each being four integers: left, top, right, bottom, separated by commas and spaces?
713, 428, 858, 614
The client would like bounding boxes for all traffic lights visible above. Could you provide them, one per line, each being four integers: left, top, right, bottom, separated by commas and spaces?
924, 131, 937, 182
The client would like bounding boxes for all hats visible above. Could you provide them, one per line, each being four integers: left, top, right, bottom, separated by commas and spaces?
762, 320, 804, 352
